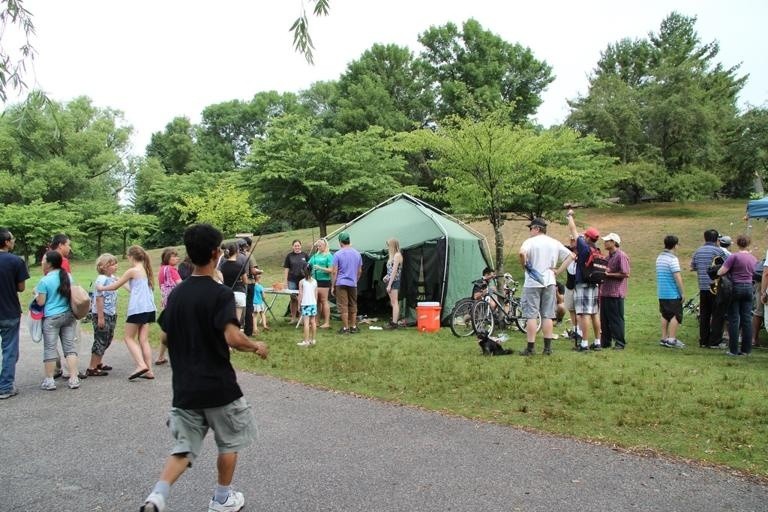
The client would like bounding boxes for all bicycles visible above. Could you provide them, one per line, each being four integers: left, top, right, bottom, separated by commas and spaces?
448, 275, 542, 338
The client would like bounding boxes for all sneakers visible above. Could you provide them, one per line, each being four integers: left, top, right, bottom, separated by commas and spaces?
386, 319, 399, 329
294, 338, 318, 346
208, 491, 246, 512
40, 363, 112, 391
0, 380, 18, 399
521, 338, 752, 360
338, 325, 362, 335
139, 493, 166, 512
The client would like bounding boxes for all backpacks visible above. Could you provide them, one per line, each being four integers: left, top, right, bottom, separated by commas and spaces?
580, 246, 608, 285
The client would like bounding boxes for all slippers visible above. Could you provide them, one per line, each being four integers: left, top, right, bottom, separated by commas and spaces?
128, 367, 154, 382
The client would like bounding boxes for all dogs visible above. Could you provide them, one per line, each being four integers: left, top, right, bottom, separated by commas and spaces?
476, 330, 514, 355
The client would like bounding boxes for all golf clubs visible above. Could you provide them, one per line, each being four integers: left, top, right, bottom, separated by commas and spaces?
572, 291, 581, 347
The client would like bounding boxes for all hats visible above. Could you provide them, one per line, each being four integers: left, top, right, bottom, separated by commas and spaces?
600, 233, 620, 245
581, 228, 598, 241
338, 232, 350, 241
721, 236, 733, 246
526, 219, 547, 229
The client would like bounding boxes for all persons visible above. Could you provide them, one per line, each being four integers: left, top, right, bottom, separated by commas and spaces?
307, 237, 333, 329
688, 225, 768, 355
0, 226, 25, 400
655, 234, 687, 349
32, 251, 86, 390
332, 233, 363, 334
282, 240, 309, 325
517, 207, 630, 354
381, 238, 403, 330
87, 236, 272, 382
298, 263, 320, 345
40, 232, 89, 381
471, 267, 505, 321
137, 222, 270, 511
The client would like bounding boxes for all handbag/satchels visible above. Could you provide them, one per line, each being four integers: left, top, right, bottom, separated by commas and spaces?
27, 297, 43, 342
68, 284, 91, 319
160, 265, 178, 309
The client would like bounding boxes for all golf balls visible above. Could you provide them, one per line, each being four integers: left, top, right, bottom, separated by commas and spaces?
730, 223, 733, 227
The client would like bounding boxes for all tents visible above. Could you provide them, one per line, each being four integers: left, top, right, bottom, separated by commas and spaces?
308, 191, 494, 324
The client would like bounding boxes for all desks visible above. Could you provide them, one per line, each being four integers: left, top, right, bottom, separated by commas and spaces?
261, 284, 320, 329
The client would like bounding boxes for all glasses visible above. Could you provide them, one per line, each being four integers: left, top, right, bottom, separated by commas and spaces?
529, 227, 532, 231
